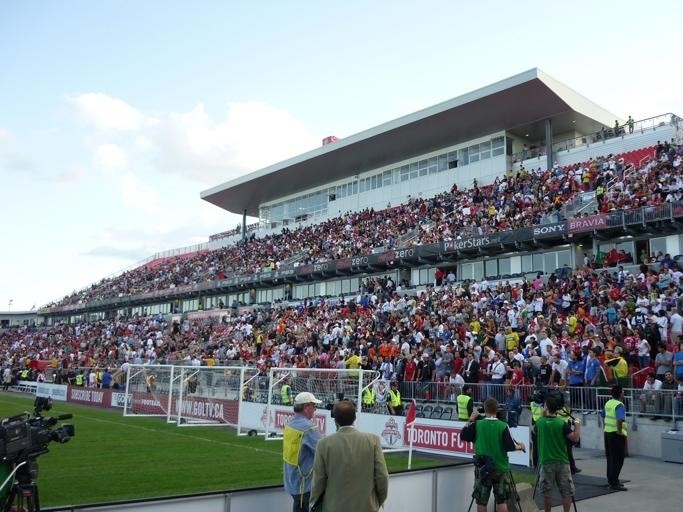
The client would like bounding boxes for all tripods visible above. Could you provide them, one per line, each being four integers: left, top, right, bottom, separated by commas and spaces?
6, 481, 42, 512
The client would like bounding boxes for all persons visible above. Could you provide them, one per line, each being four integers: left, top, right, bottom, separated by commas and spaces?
599, 385, 627, 492
531, 397, 581, 512
528, 389, 545, 470
555, 391, 582, 475
460, 397, 525, 512
310, 399, 388, 512
280, 391, 322, 512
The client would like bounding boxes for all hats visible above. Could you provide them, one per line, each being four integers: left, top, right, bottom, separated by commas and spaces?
294, 391, 323, 405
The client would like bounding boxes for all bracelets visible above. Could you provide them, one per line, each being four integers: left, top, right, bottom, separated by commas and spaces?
518, 443, 522, 450
